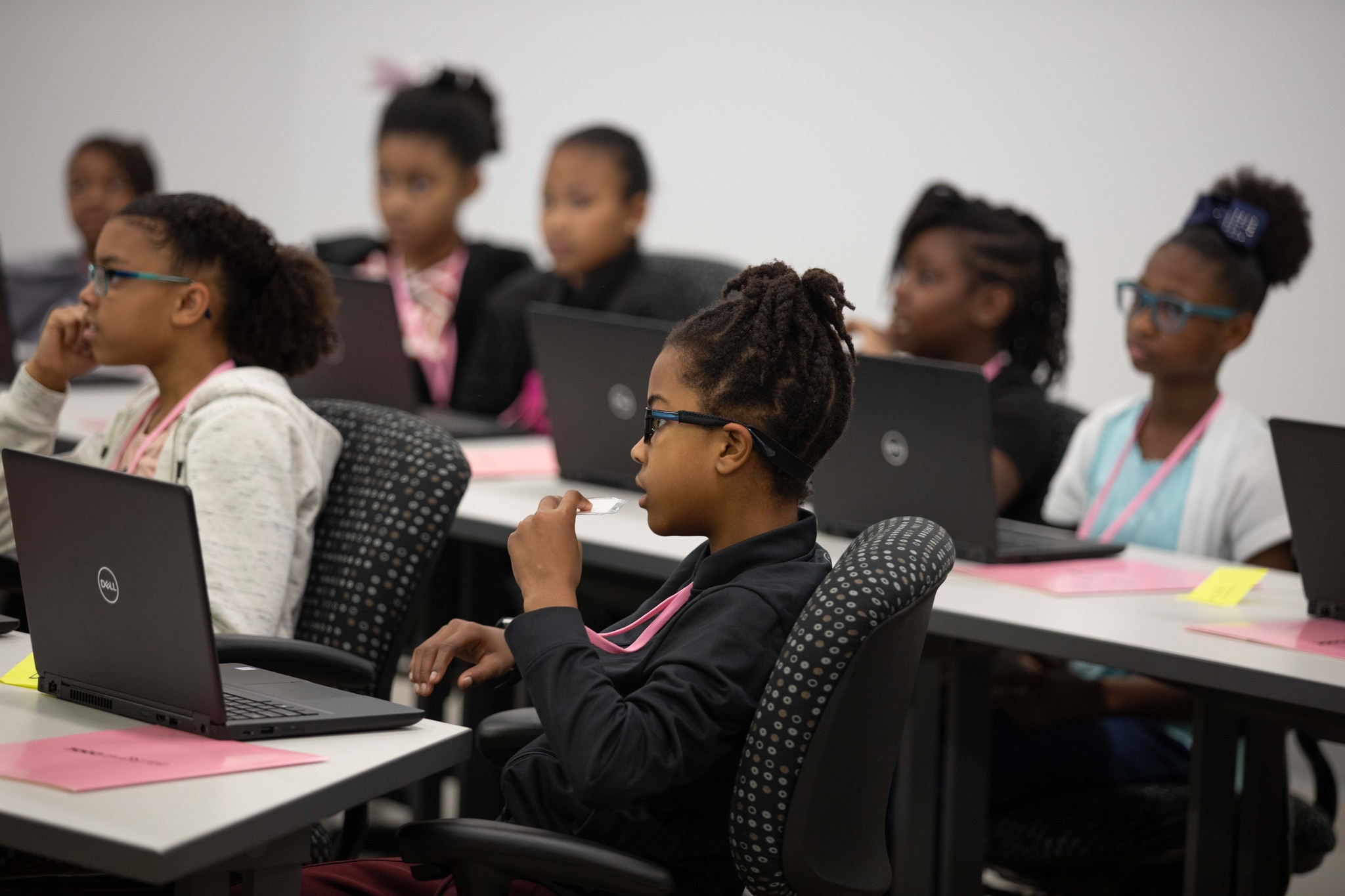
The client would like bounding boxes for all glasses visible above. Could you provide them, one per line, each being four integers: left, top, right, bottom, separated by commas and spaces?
644, 405, 779, 469
1117, 282, 1238, 335
86, 262, 212, 321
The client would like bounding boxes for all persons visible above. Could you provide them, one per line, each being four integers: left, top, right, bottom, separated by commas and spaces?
0, 193, 343, 642
40, 64, 1315, 896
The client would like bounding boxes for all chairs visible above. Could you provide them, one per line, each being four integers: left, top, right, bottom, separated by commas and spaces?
399, 517, 954, 896
209, 396, 469, 696
1086, 702, 1339, 896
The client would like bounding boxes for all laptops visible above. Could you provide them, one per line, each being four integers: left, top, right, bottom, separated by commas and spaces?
519, 298, 715, 492
812, 348, 1128, 567
282, 273, 527, 439
1, 258, 146, 387
1269, 418, 1345, 620
0, 443, 428, 742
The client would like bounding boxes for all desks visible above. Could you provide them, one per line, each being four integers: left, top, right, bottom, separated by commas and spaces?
450, 430, 1345, 895
0, 627, 472, 896
0, 376, 150, 447
